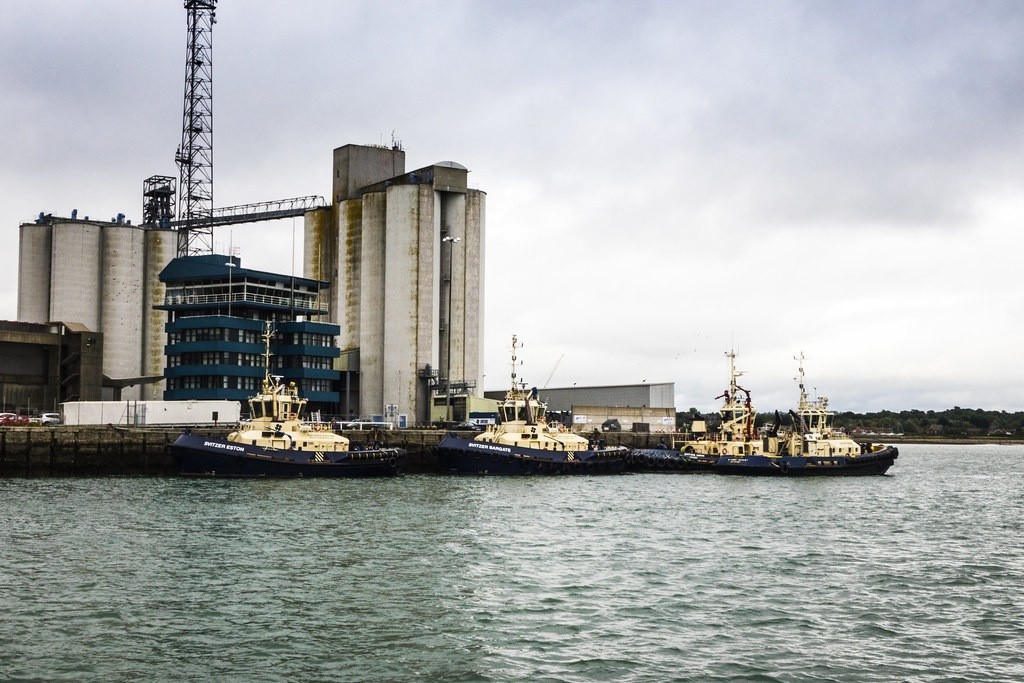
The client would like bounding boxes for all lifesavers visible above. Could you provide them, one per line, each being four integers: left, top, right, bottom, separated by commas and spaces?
538, 416, 546, 424
743, 428, 748, 434
722, 448, 727, 455
314, 424, 321, 432
559, 426, 565, 433
845, 453, 850, 459
788, 432, 792, 438
289, 412, 296, 420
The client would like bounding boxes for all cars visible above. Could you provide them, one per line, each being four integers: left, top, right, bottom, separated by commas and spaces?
0, 412, 26, 426
30, 412, 63, 426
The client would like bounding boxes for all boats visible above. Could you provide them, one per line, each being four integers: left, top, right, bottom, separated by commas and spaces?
436, 336, 627, 472
167, 316, 407, 474
631, 328, 900, 475
